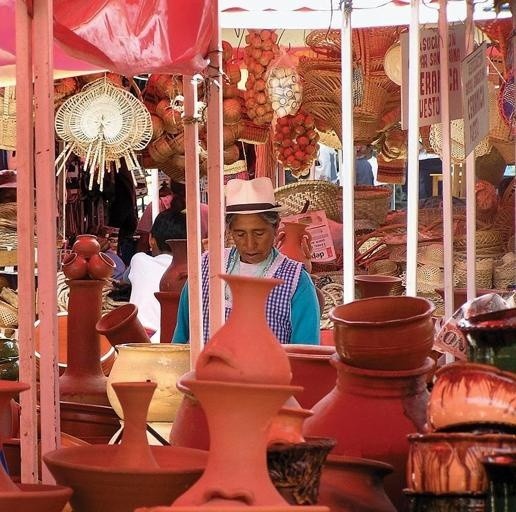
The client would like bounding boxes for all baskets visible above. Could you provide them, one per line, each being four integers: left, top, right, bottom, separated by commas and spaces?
489, 108, 516, 165
0, 76, 64, 152
140, 41, 268, 181
337, 186, 391, 225
272, 181, 337, 218
301, 26, 404, 142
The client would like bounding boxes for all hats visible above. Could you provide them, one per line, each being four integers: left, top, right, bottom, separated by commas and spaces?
417, 244, 457, 267
368, 259, 397, 275
224, 177, 282, 214
399, 265, 459, 293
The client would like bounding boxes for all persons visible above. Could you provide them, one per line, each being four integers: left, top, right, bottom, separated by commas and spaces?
171, 176, 320, 345
123, 208, 186, 343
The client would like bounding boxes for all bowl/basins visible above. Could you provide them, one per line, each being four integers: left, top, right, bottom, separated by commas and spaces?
455, 307, 516, 348
